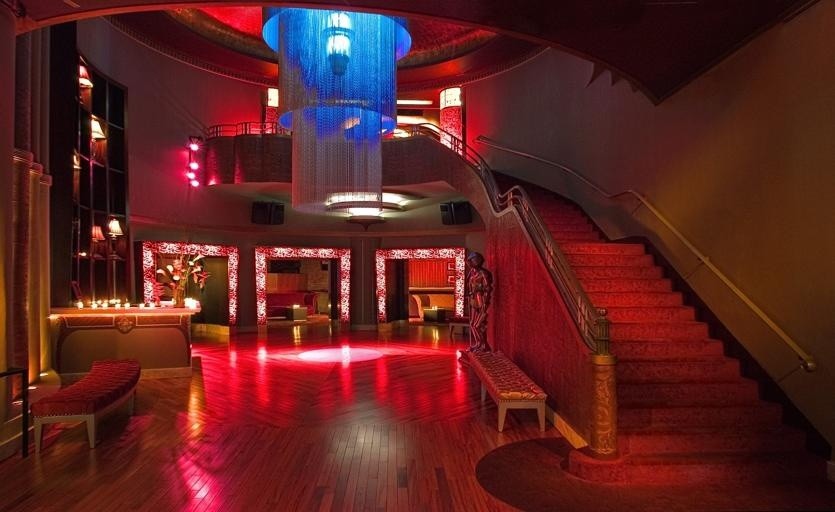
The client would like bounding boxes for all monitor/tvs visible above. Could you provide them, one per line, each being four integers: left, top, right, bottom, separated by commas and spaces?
452, 202, 473, 226
252, 202, 285, 228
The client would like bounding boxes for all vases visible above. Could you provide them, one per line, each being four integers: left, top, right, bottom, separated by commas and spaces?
172, 285, 187, 309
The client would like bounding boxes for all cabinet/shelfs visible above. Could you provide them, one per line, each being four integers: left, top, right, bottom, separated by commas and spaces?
69, 50, 133, 307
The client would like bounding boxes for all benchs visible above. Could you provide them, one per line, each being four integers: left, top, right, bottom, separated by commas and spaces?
29, 357, 141, 456
468, 348, 549, 435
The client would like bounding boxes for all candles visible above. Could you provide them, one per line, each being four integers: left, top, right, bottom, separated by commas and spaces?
101, 300, 107, 310
150, 301, 156, 310
75, 301, 85, 311
184, 296, 202, 310
90, 300, 98, 310
158, 300, 173, 308
114, 301, 121, 311
138, 302, 145, 309
123, 302, 131, 312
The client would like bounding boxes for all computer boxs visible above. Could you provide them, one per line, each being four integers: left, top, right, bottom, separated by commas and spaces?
439, 204, 452, 225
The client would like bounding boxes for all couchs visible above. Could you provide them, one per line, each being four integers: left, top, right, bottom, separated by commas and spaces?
265, 290, 320, 317
407, 294, 420, 319
413, 293, 434, 318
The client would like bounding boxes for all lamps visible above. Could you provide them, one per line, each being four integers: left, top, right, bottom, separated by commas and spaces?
106, 217, 126, 242
91, 223, 107, 255
78, 64, 93, 103
261, 5, 412, 221
90, 116, 108, 159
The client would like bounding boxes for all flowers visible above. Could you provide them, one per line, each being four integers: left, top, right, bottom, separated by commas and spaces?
156, 252, 211, 292
